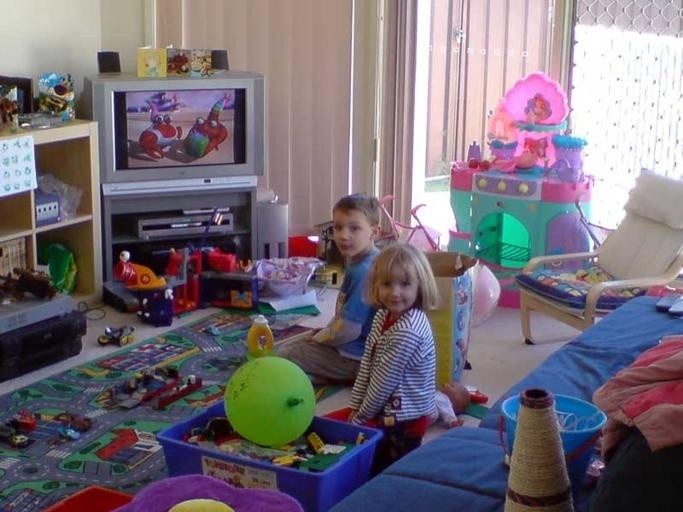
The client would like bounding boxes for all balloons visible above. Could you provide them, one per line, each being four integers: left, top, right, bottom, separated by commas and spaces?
222, 353, 318, 446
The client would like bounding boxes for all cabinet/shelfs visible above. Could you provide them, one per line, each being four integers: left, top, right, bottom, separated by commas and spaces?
102, 176, 257, 282
0, 119, 105, 319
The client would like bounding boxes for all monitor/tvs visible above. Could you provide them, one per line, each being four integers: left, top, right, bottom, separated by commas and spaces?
80, 69, 264, 197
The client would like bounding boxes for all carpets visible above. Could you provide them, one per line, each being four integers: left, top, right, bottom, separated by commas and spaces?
1, 304, 327, 512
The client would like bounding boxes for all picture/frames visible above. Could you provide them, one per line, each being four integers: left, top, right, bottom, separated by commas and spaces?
138, 49, 167, 79
0, 76, 33, 124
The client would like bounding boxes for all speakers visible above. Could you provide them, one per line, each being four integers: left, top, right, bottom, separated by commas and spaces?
97, 51, 120, 73
211, 50, 228, 70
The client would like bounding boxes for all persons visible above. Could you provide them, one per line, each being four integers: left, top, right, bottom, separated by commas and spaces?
0, 81, 19, 124
277, 192, 387, 386
342, 240, 443, 479
431, 383, 473, 428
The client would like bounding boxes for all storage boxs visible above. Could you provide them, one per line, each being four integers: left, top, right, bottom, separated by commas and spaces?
156, 398, 384, 512
419, 250, 477, 392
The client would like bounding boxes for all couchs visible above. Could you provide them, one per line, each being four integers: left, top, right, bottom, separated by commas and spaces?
328, 296, 682, 512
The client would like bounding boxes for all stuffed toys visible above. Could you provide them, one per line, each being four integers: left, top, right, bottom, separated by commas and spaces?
38, 76, 74, 117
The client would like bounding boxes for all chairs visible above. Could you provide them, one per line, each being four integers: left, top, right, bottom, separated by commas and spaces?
515, 169, 682, 346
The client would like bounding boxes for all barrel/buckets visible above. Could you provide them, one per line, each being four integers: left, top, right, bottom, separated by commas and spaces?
496, 391, 609, 474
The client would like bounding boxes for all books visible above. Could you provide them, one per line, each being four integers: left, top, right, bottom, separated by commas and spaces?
0, 237, 28, 282
